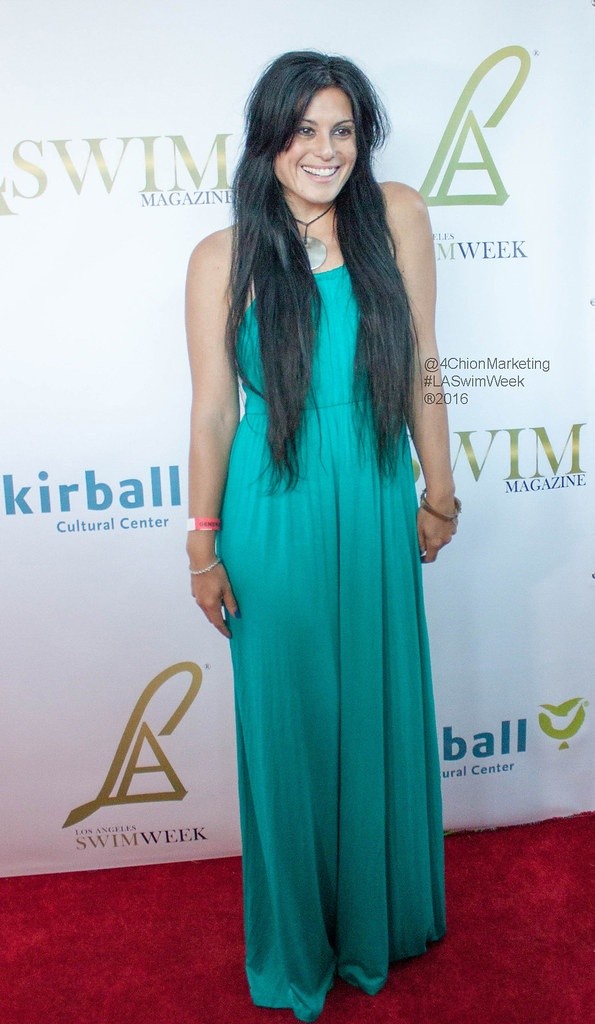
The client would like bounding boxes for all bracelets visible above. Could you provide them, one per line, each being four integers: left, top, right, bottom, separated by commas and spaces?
189, 557, 221, 574
419, 488, 463, 521
186, 517, 221, 530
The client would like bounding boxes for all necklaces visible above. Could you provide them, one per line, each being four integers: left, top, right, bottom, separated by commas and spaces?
294, 201, 336, 270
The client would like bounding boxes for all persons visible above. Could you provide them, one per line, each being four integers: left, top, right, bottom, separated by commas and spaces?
186, 49, 458, 1024
